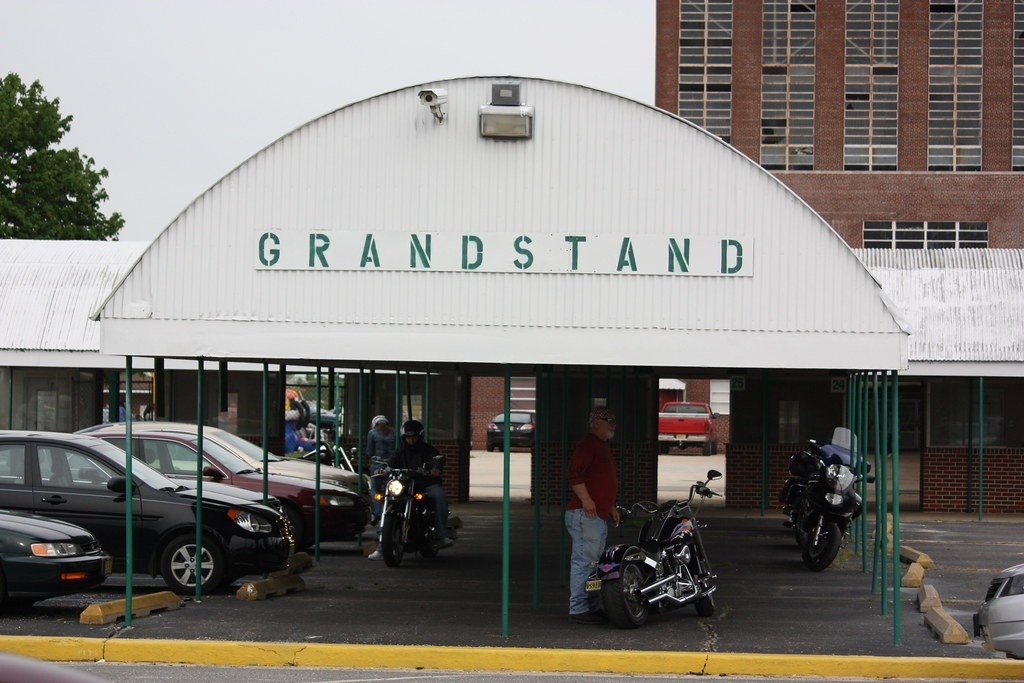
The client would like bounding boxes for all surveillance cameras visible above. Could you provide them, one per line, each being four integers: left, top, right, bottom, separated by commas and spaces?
418, 89, 447, 106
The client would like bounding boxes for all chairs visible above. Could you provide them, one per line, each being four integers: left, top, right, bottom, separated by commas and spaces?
146, 448, 156, 465
48, 455, 70, 486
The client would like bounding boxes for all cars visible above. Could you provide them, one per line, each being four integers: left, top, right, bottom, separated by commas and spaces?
0, 430, 295, 596
72, 421, 374, 552
0, 508, 114, 615
486, 410, 536, 452
973, 564, 1024, 659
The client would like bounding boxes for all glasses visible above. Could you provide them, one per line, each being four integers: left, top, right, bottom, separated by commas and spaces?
596, 415, 617, 425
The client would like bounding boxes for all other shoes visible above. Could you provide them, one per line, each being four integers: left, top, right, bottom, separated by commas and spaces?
367, 549, 384, 561
438, 537, 454, 548
569, 608, 608, 625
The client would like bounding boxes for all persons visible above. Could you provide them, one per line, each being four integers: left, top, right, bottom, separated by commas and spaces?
565, 406, 619, 626
367, 413, 396, 526
368, 420, 454, 561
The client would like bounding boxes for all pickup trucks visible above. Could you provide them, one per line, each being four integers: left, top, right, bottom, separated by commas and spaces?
658, 401, 721, 456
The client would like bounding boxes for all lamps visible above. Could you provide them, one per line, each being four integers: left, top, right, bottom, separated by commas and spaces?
476, 78, 534, 141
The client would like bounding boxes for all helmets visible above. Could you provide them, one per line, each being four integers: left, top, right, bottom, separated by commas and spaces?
371, 415, 390, 430
401, 420, 425, 450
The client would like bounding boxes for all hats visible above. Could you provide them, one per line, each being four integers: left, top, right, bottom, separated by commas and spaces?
589, 405, 613, 423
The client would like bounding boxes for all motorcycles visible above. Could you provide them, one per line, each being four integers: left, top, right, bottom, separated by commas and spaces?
778, 427, 876, 572
585, 469, 723, 628
369, 456, 447, 567
